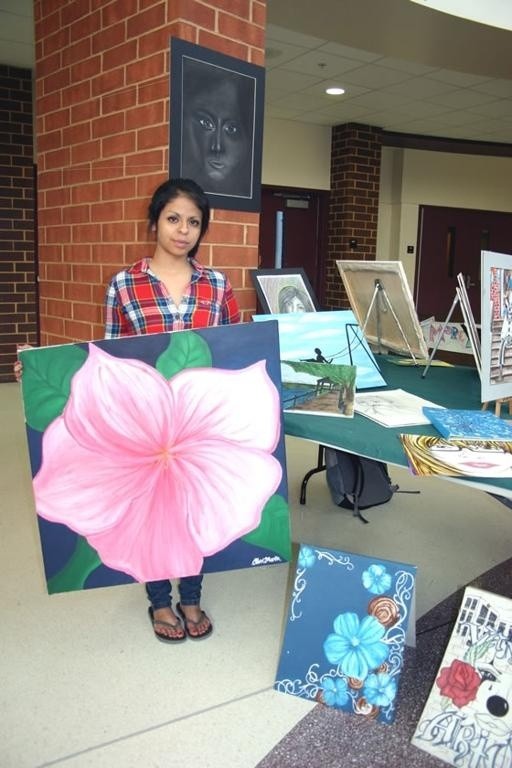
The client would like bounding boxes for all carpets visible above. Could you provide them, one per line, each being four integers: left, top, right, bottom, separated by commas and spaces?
252, 555, 512, 768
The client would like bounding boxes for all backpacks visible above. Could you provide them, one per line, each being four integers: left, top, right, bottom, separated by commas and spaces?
325, 447, 422, 524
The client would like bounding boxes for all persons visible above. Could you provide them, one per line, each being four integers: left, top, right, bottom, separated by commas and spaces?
15, 177, 241, 645
398, 433, 511, 478
184, 75, 252, 197
279, 287, 316, 314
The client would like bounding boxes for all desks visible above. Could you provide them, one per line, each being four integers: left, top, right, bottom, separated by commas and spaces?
284, 355, 512, 518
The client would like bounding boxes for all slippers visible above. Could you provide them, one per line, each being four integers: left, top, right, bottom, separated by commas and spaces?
176, 601, 213, 641
149, 606, 187, 644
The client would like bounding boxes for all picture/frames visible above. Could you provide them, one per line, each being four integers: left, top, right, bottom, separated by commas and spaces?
481, 250, 511, 402
249, 266, 323, 315
337, 260, 430, 360
